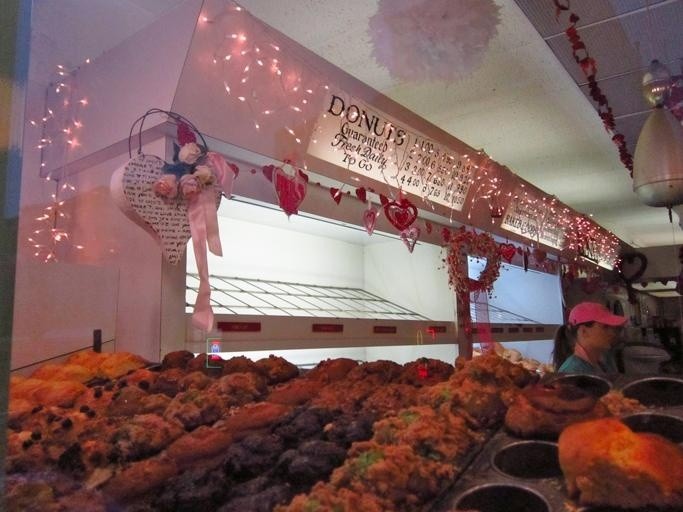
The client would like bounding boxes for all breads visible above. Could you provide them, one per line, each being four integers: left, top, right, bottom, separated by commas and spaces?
557, 418, 682, 512
8, 350, 596, 512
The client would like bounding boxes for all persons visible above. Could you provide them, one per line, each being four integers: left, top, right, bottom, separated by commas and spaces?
545, 301, 629, 389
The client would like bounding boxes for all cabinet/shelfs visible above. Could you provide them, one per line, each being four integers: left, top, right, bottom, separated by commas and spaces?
19, 189, 564, 367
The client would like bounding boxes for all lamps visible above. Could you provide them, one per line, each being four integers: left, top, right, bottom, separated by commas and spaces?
633, 58, 682, 208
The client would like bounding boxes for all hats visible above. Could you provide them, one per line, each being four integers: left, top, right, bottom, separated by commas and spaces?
568, 302, 628, 326
